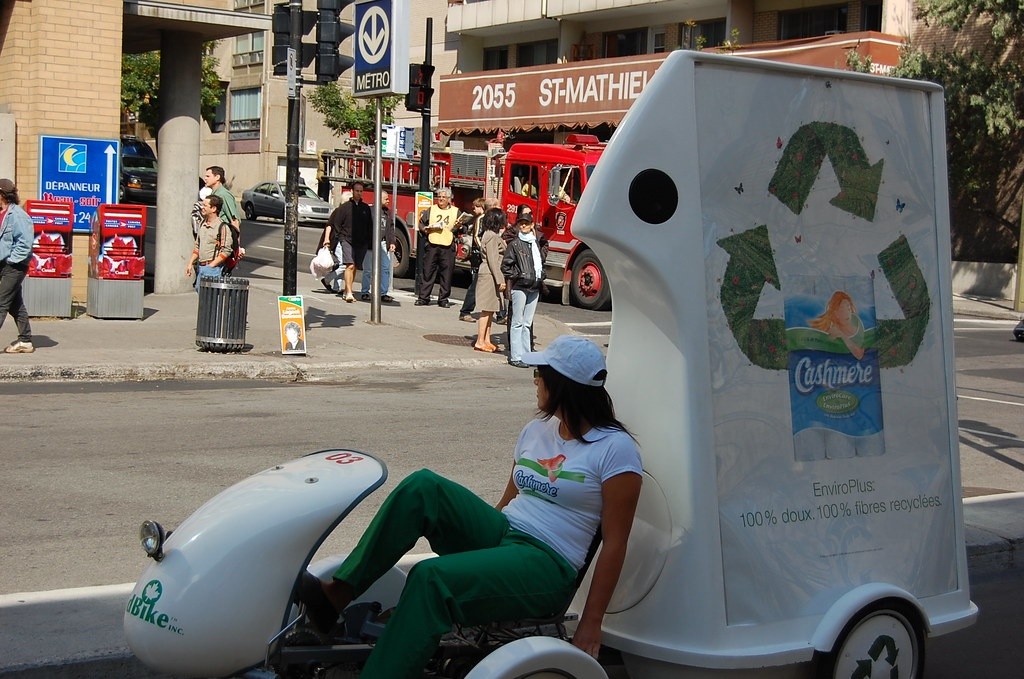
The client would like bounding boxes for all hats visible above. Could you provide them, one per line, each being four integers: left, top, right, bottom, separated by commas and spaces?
516, 213, 532, 222
521, 333, 607, 386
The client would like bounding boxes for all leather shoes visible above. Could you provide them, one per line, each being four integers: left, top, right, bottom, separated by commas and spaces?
414, 298, 428, 305
438, 300, 450, 308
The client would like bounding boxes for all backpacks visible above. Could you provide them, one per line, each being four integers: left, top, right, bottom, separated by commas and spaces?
201, 219, 239, 276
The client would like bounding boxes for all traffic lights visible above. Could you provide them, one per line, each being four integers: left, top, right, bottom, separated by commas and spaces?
315, 0, 355, 82
300, 10, 317, 67
405, 63, 435, 110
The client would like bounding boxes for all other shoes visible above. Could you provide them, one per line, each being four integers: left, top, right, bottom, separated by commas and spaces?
381, 295, 393, 302
343, 293, 356, 301
498, 316, 508, 324
510, 360, 529, 368
321, 278, 332, 290
362, 294, 370, 300
331, 289, 339, 294
346, 293, 353, 301
292, 570, 339, 629
460, 314, 476, 322
5, 340, 34, 353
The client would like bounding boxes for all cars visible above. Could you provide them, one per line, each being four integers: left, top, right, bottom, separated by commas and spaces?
240, 181, 329, 227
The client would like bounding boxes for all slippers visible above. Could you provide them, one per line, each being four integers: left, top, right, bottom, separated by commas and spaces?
474, 344, 492, 352
488, 344, 502, 352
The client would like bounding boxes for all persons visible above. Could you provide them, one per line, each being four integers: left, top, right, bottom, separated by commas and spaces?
199, 166, 244, 260
316, 182, 373, 302
459, 197, 549, 368
185, 195, 233, 292
414, 188, 468, 308
294, 336, 643, 679
285, 322, 304, 350
361, 189, 396, 302
0, 179, 34, 353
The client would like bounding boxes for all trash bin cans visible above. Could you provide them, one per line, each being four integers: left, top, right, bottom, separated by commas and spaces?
195, 275, 249, 353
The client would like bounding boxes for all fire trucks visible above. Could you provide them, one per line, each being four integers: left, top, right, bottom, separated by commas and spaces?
320, 132, 612, 312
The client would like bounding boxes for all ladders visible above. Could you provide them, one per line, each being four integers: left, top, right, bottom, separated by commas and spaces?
316, 148, 450, 193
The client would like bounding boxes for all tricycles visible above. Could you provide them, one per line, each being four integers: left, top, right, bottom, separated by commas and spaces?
121, 447, 613, 679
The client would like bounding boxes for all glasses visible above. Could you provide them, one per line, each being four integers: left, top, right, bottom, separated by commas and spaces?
533, 368, 541, 376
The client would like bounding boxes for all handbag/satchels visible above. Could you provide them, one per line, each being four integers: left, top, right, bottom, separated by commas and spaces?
309, 245, 339, 281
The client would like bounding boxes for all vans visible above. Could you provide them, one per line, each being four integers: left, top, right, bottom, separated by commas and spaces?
119, 133, 158, 204
